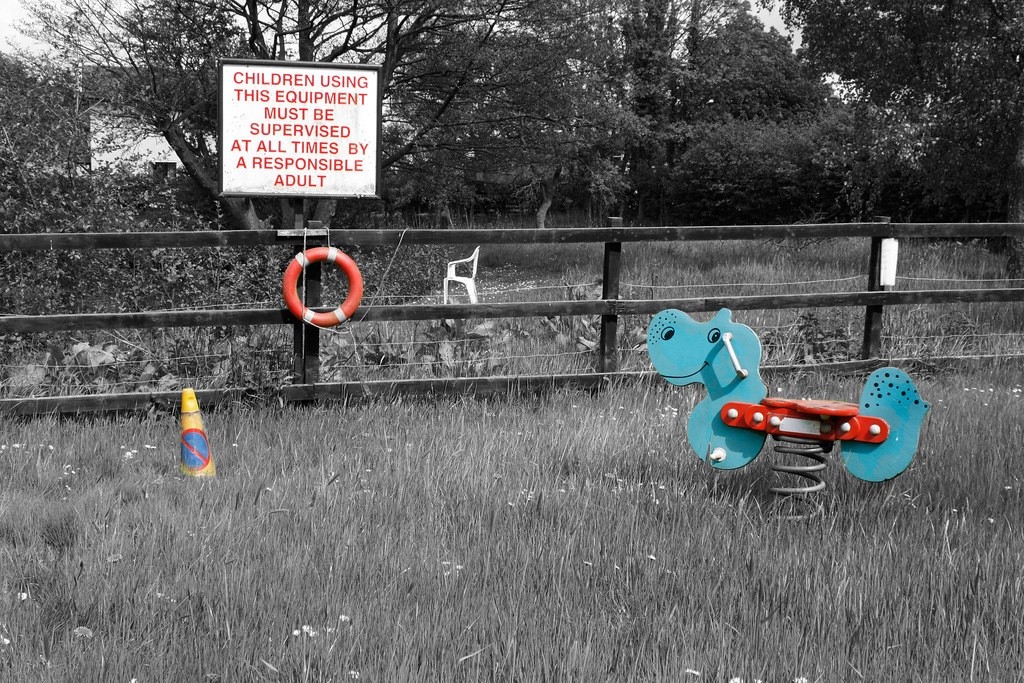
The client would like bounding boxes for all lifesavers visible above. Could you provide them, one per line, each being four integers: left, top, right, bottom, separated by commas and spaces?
282, 246, 363, 327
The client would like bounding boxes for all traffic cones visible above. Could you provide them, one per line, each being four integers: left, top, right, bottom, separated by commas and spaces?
178, 386, 221, 482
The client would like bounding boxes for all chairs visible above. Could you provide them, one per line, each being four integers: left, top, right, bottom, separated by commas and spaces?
443, 245, 481, 304
